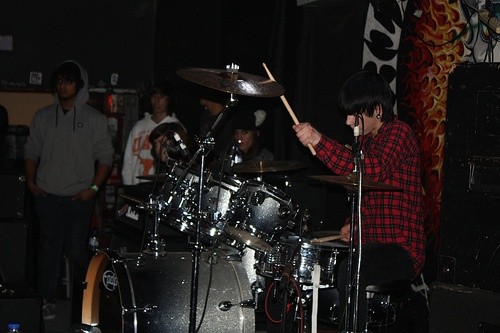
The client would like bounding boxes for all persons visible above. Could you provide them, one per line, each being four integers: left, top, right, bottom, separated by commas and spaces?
123, 79, 186, 252
293, 69, 425, 333
24, 61, 115, 333
200, 98, 233, 164
233, 111, 279, 162
149, 122, 200, 176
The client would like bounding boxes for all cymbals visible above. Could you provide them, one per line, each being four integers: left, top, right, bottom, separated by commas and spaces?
175, 66, 285, 98
233, 162, 311, 174
306, 175, 401, 192
137, 174, 168, 179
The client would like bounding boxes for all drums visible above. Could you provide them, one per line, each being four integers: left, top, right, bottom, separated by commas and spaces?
81, 247, 256, 333
214, 228, 356, 290
227, 180, 311, 255
150, 160, 200, 235
188, 173, 244, 253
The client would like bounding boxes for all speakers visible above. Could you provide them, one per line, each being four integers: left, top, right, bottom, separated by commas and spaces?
427, 283, 500, 332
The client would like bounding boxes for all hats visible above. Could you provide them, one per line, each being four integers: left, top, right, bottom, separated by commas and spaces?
230, 112, 265, 131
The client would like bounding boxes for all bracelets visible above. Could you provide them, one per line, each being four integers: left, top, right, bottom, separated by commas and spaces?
91, 184, 98, 192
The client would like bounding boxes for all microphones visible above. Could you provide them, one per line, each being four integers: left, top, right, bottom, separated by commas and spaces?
87, 227, 100, 256
354, 116, 360, 145
174, 132, 190, 159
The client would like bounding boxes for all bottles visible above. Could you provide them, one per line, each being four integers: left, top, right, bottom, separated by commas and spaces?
9, 325, 19, 333
